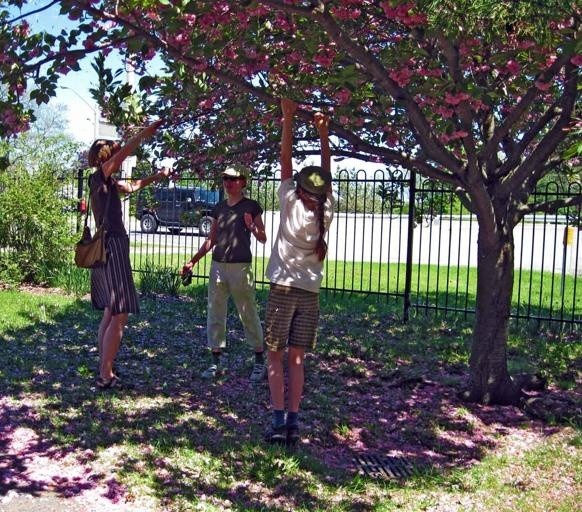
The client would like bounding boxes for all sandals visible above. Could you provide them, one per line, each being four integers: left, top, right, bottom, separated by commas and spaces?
96, 375, 136, 390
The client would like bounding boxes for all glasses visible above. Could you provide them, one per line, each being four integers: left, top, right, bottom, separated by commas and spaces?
221, 175, 237, 181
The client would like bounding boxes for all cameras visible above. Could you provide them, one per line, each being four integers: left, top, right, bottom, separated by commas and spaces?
181, 266, 192, 286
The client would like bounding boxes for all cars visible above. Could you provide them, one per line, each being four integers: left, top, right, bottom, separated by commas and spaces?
47, 182, 87, 215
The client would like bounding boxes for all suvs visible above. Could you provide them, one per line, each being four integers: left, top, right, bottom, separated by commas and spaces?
136, 186, 220, 236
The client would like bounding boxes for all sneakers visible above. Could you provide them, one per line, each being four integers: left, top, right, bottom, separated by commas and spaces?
200, 364, 218, 378
265, 422, 286, 440
250, 363, 266, 380
287, 424, 300, 439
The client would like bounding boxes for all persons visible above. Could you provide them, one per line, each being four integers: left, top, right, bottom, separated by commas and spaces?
92, 117, 175, 391
177, 164, 267, 382
264, 97, 335, 442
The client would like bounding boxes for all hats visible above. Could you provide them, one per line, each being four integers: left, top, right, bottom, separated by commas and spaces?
294, 166, 332, 195
221, 163, 250, 177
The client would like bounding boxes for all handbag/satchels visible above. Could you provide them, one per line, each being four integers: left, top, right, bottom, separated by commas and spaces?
75, 225, 107, 268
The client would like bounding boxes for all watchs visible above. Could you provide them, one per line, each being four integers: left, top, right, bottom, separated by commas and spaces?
249, 222, 256, 230
